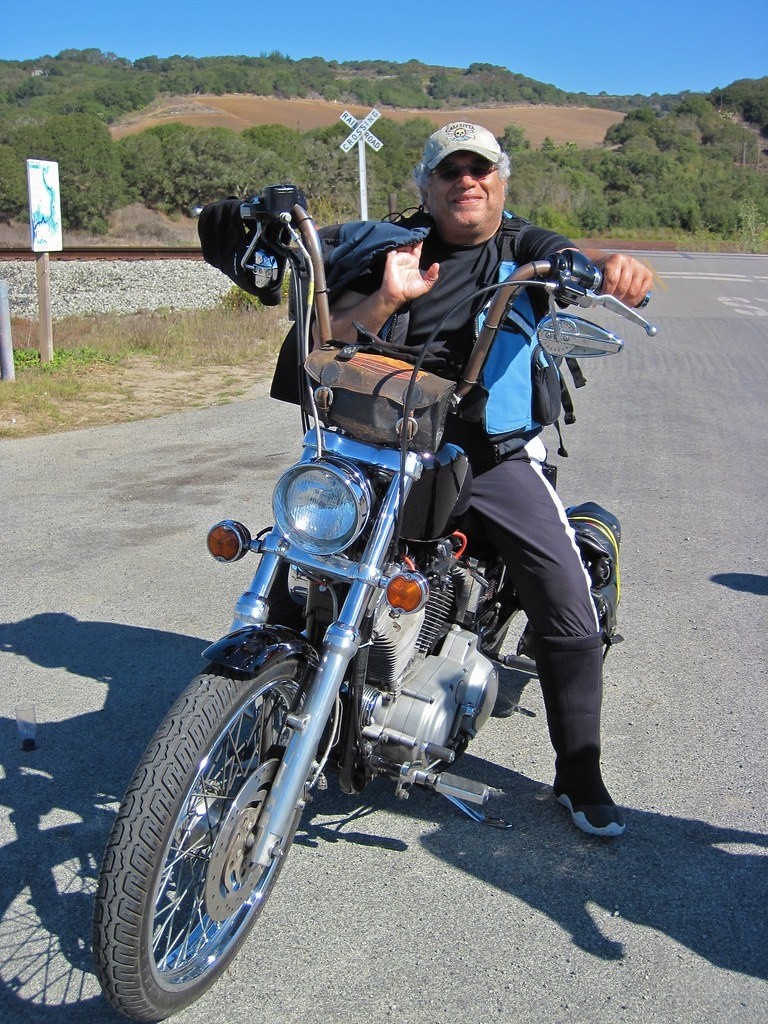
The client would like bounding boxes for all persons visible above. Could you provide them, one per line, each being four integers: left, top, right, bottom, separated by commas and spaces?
298, 123, 629, 839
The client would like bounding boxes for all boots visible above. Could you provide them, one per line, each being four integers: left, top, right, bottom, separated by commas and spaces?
531, 632, 626, 837
226, 746, 263, 770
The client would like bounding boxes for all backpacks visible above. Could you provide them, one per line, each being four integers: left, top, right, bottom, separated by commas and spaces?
516, 502, 624, 660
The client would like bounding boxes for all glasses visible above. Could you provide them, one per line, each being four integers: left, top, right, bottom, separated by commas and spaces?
428, 158, 498, 183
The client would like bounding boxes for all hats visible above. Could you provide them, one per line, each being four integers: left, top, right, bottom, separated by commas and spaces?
422, 122, 501, 169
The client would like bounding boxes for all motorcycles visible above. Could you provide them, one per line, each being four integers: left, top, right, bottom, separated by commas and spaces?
92, 182, 656, 1024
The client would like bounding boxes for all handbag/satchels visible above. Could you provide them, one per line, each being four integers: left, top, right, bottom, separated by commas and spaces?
500, 217, 587, 426
